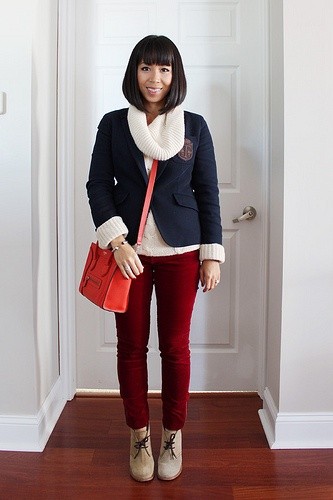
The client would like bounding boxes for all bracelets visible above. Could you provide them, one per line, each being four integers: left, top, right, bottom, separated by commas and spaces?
110, 240, 128, 253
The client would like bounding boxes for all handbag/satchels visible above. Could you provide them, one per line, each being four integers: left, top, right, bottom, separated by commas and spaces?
79, 241, 138, 313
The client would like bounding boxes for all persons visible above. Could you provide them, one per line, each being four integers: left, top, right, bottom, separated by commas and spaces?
86, 34, 226, 482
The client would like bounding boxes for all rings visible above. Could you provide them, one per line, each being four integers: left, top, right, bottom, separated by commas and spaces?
124, 266, 130, 271
214, 280, 220, 285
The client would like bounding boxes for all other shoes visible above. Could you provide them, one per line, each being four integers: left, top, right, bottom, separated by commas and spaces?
130, 420, 182, 482
